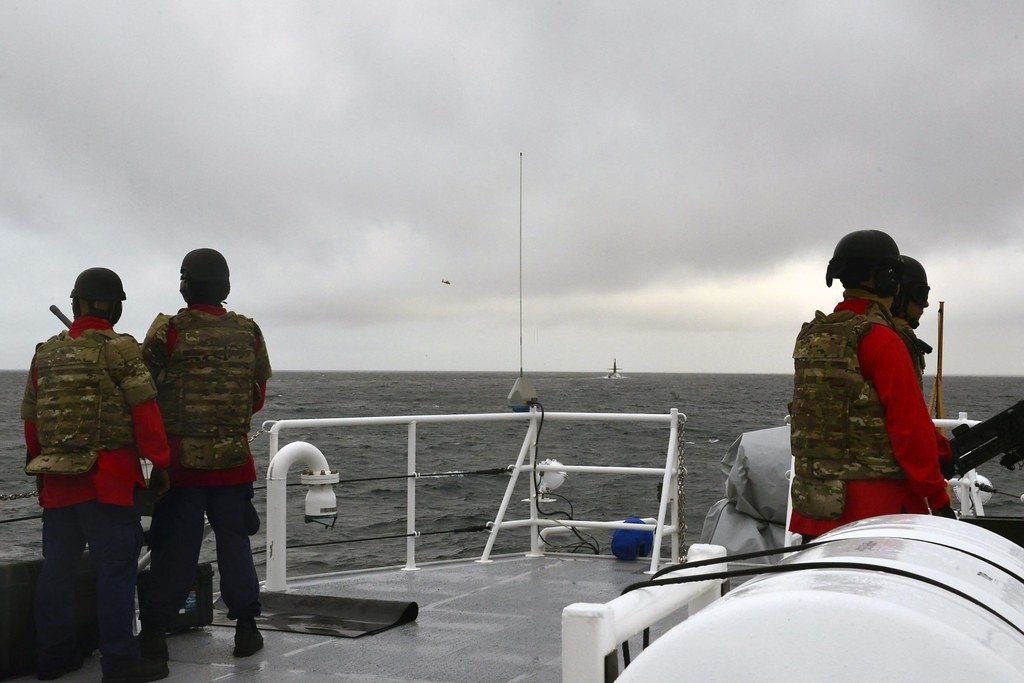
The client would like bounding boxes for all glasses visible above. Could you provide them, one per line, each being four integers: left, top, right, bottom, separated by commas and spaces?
906, 284, 930, 305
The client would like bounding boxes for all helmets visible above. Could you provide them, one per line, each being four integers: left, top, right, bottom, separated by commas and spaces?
825, 230, 901, 287
70, 267, 126, 302
900, 255, 932, 307
180, 248, 230, 281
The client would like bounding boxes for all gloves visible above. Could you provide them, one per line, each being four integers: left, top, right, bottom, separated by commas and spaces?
941, 464, 956, 481
932, 502, 957, 520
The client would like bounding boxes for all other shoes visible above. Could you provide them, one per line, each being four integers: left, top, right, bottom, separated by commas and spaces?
38, 655, 83, 680
132, 619, 170, 663
232, 618, 264, 657
102, 657, 169, 683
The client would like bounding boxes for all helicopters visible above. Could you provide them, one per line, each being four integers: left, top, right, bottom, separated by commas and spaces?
442, 280, 450, 284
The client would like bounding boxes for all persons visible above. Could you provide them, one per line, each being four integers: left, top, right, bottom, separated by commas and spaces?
788, 230, 957, 545
135, 248, 272, 658
889, 255, 933, 392
19, 268, 171, 683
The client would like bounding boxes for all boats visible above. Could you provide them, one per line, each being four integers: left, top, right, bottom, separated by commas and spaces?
607, 358, 623, 379
